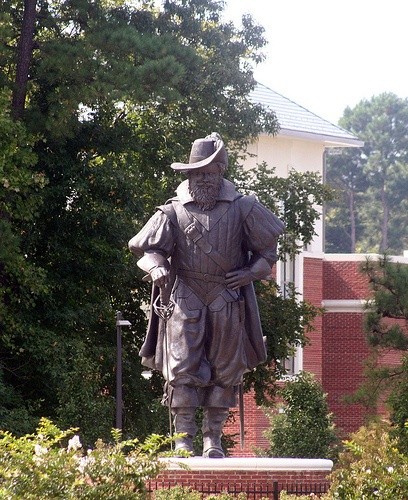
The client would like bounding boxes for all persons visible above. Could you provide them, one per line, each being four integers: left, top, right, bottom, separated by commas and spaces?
127, 131, 286, 458
